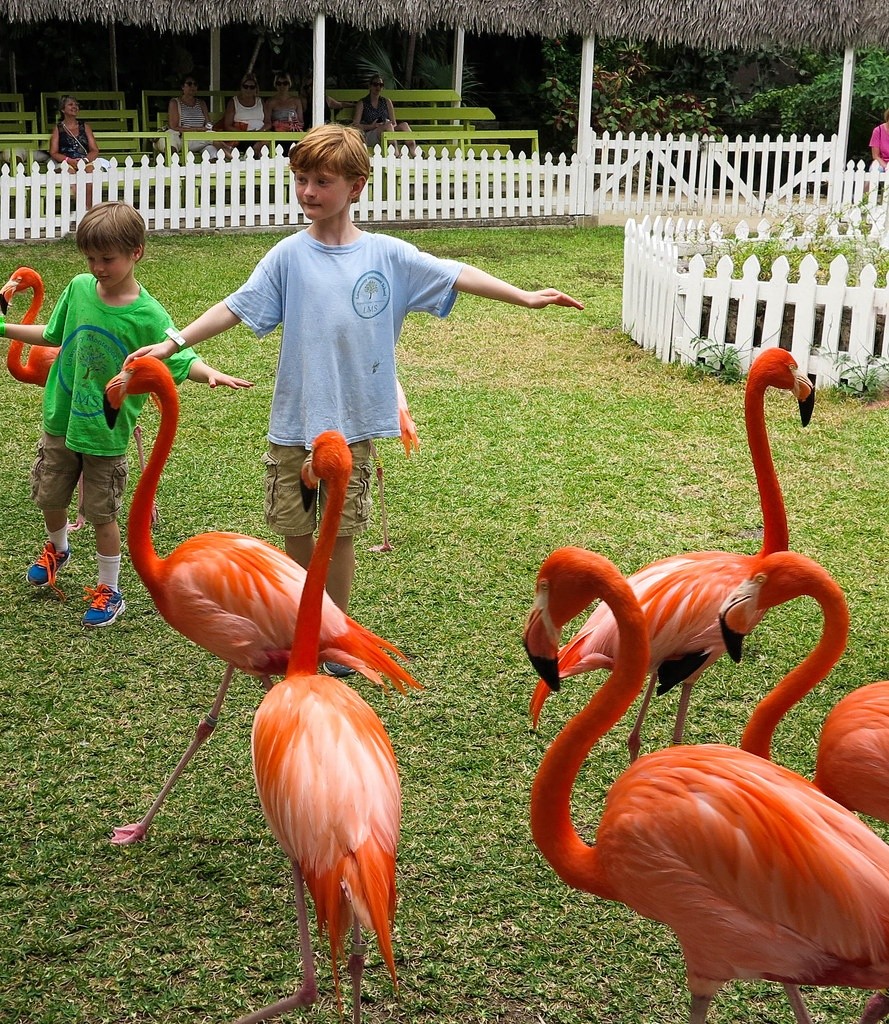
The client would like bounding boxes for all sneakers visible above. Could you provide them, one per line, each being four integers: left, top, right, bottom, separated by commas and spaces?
79, 584, 127, 628
26, 541, 71, 604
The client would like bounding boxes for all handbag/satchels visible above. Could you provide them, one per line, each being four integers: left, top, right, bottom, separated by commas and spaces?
151, 97, 182, 154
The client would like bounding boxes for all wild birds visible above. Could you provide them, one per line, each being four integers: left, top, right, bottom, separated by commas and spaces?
522, 348, 889, 1024
0, 265, 427, 1024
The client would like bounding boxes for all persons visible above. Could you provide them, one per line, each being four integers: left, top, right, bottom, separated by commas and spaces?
0, 201, 255, 627
352, 74, 425, 160
49, 96, 99, 210
122, 123, 585, 677
863, 109, 889, 193
165, 72, 357, 164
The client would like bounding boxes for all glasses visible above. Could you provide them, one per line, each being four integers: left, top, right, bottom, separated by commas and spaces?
242, 84, 256, 90
184, 82, 198, 87
371, 82, 384, 87
276, 81, 289, 86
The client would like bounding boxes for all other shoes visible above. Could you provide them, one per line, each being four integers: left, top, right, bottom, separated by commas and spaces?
323, 661, 355, 678
413, 150, 424, 159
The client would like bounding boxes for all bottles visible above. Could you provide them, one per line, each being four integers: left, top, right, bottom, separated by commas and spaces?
385, 117, 390, 123
288, 110, 294, 122
205, 123, 212, 132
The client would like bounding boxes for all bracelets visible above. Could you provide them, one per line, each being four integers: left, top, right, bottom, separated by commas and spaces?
65, 157, 69, 161
0, 315, 6, 337
83, 158, 89, 162
165, 328, 186, 353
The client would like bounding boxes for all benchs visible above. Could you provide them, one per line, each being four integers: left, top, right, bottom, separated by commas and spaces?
142, 90, 312, 218
325, 86, 554, 214
0, 89, 184, 229
